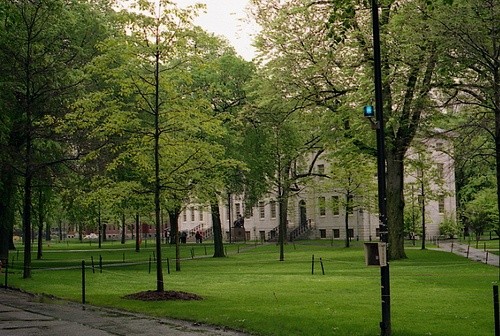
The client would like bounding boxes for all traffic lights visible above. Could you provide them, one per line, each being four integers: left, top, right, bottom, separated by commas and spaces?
364, 106, 374, 117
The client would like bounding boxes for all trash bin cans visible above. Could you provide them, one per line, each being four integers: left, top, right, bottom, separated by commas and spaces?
364, 241, 379, 266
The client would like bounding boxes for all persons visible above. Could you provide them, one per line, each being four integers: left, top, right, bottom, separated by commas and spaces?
162, 229, 202, 244
46, 235, 51, 247
231, 214, 244, 229
